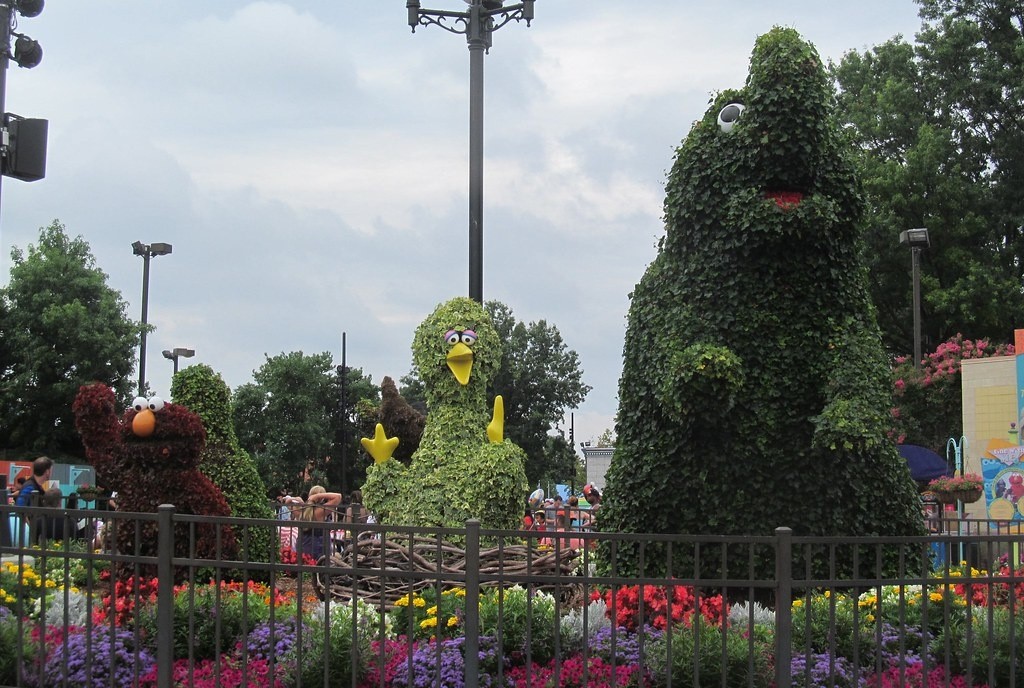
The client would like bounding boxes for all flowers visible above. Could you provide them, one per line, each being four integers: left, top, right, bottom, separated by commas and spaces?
929, 473, 984, 492
78, 484, 98, 492
921, 492, 935, 502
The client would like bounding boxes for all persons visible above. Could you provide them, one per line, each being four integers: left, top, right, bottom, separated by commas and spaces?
6, 456, 121, 549
520, 483, 609, 552
267, 485, 384, 560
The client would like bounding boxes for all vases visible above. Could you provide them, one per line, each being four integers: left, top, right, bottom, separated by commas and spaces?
936, 493, 957, 504
80, 493, 97, 501
957, 491, 981, 503
